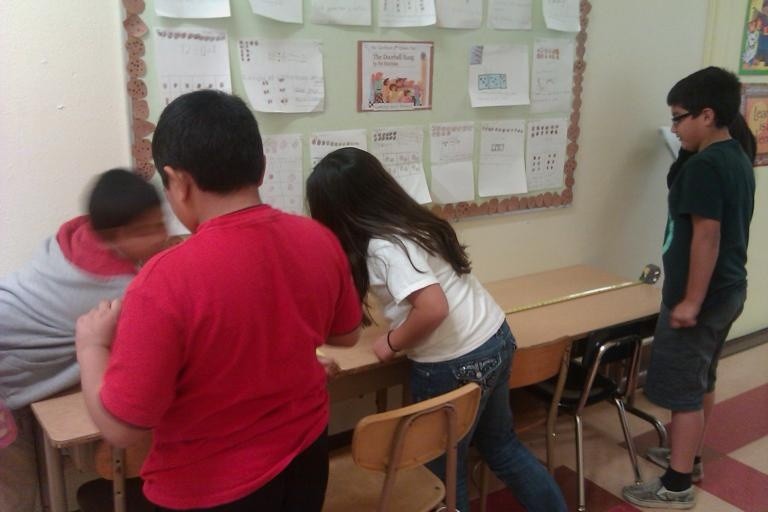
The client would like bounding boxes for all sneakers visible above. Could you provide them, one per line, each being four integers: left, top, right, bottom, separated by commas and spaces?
645, 447, 704, 482
622, 475, 696, 510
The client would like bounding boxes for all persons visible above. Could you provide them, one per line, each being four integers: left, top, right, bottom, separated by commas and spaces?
0, 167, 185, 512
656, 111, 758, 188
300, 142, 567, 511
621, 64, 758, 510
72, 87, 367, 510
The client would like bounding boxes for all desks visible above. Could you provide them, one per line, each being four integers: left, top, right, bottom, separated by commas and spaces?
3, 262, 669, 511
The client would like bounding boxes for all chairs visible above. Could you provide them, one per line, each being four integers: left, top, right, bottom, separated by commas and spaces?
278, 382, 486, 512
90, 427, 157, 510
495, 335, 574, 486
524, 313, 646, 512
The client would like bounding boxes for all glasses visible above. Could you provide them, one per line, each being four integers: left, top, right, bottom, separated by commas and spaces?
670, 108, 705, 125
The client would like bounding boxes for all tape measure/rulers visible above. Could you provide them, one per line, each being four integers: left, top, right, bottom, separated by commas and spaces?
504, 264, 661, 314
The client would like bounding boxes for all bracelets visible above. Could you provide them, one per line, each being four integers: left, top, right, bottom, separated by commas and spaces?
386, 326, 401, 356
